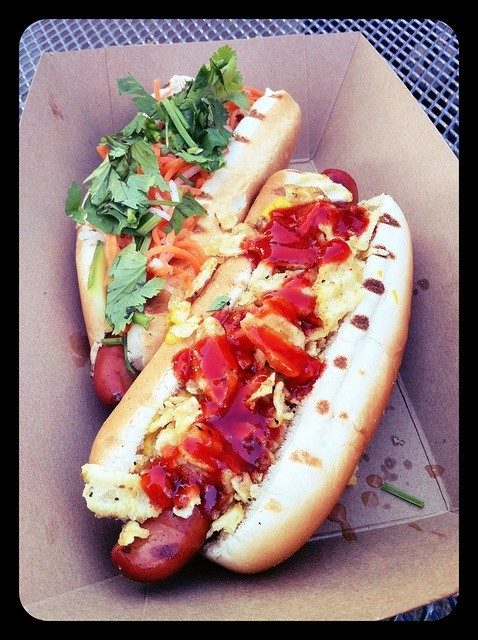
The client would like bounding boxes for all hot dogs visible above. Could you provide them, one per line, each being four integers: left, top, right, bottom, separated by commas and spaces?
75, 166, 414, 579
74, 75, 301, 401
